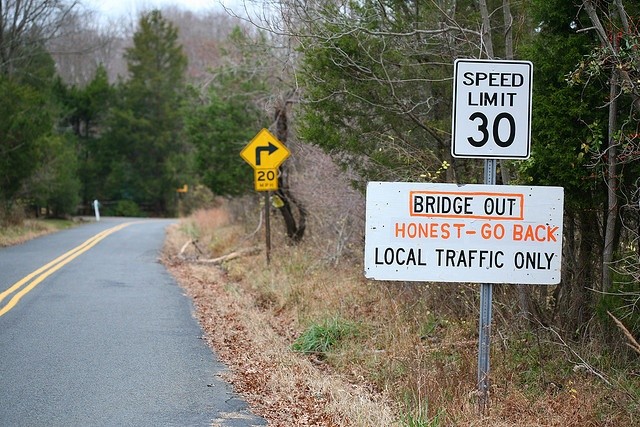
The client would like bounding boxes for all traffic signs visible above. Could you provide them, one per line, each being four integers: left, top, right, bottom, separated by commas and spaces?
451, 59, 533, 160
